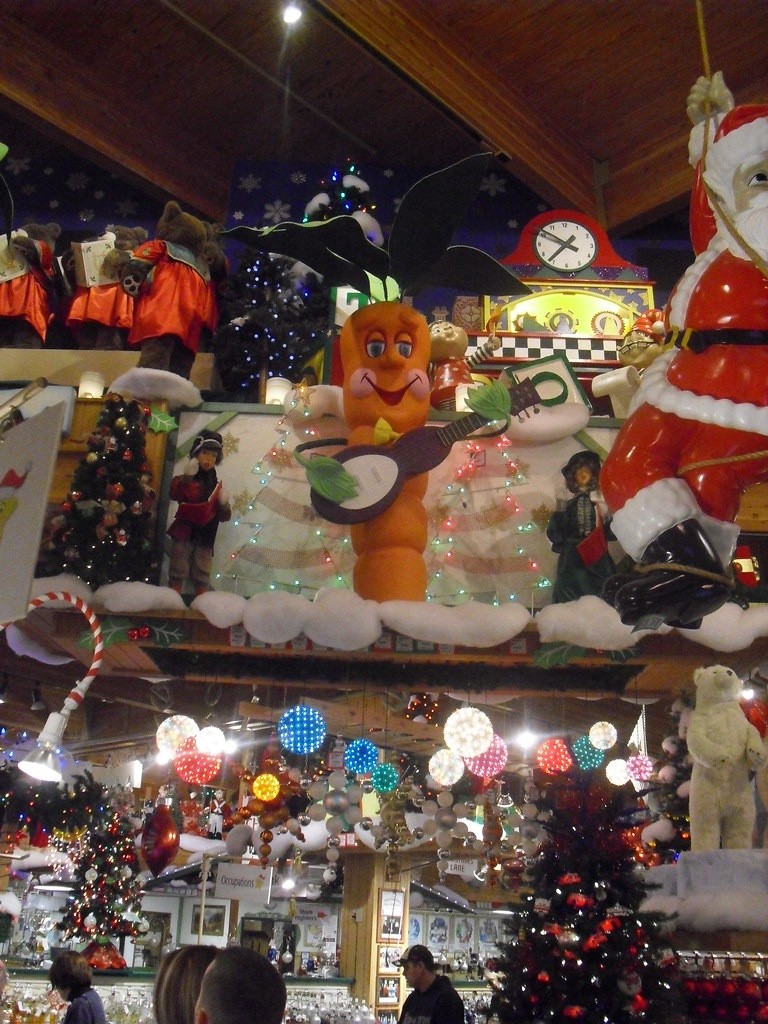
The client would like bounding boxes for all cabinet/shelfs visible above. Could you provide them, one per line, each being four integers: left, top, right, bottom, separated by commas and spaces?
448, 980, 493, 1024
677, 951, 768, 1024
0, 343, 224, 592
8, 968, 357, 1024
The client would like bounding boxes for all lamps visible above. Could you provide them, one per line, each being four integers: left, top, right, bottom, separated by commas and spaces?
0, 682, 9, 703
31, 688, 46, 710
77, 371, 107, 399
0, 591, 106, 784
266, 377, 293, 405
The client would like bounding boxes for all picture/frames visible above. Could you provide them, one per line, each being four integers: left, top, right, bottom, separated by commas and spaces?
306, 955, 375, 1024
376, 976, 401, 1006
376, 887, 408, 944
191, 904, 225, 937
377, 945, 402, 975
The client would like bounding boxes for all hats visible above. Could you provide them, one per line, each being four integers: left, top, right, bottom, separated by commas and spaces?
392, 945, 434, 967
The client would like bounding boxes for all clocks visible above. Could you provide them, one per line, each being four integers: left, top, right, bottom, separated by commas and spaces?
530, 219, 598, 273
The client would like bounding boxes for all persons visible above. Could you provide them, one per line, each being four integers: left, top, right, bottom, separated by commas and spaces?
391, 944, 494, 1024
49, 951, 108, 1024
600, 71, 768, 626
592, 309, 667, 417
429, 320, 501, 411
547, 451, 618, 604
142, 944, 287, 1024
166, 428, 231, 596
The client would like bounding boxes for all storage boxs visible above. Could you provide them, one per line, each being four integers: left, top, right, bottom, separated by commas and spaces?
0, 231, 30, 282
70, 237, 120, 289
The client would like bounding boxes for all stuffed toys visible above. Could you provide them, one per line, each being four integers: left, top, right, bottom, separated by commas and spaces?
689, 664, 768, 851
0, 201, 229, 381
